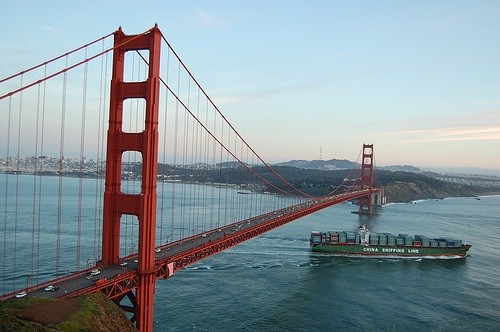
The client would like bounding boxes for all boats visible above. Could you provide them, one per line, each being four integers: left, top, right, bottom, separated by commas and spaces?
310, 231, 472, 258
237, 190, 253, 194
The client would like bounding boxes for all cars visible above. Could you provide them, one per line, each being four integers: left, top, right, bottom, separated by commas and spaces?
12, 188, 378, 300
